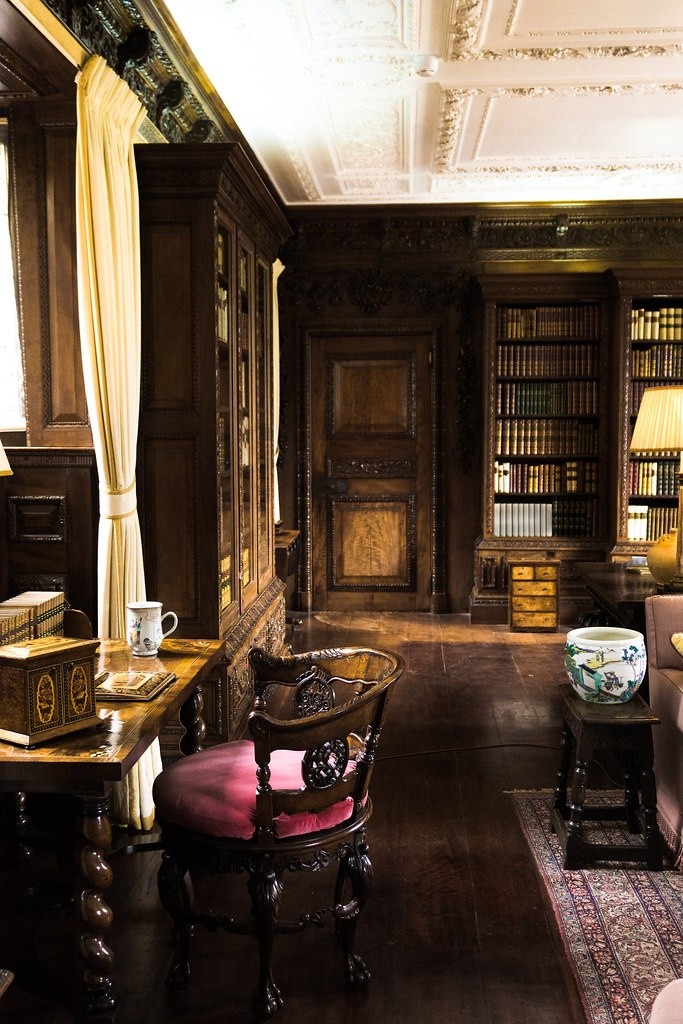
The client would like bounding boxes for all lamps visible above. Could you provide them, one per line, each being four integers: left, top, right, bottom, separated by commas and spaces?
554, 214, 569, 232
628, 384, 683, 595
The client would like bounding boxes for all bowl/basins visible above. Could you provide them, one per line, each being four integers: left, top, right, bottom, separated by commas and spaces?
563, 627, 649, 706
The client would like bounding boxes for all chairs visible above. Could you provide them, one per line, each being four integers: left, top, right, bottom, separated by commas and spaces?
151, 647, 407, 1020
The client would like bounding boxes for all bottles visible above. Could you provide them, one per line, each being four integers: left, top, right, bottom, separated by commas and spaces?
646, 527, 678, 590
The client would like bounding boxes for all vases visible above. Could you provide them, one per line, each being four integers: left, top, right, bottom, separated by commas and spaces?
563, 626, 648, 706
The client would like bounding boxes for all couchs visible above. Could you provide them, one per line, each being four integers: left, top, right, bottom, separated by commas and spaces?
645, 594, 683, 871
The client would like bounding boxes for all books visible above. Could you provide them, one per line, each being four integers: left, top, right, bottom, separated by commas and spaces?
628, 307, 683, 541
626, 555, 651, 575
1, 591, 64, 645
91, 670, 175, 701
493, 306, 600, 538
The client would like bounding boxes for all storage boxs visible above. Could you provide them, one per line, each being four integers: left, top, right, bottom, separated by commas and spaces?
0, 635, 106, 751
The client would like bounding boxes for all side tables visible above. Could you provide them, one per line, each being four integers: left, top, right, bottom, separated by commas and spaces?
547, 683, 664, 873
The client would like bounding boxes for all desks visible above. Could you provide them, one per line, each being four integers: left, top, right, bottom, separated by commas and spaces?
570, 559, 683, 635
0, 637, 228, 1024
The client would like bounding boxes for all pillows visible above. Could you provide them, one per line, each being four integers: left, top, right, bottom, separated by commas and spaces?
670, 633, 683, 658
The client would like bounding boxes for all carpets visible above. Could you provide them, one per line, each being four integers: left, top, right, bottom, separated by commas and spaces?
502, 788, 683, 1024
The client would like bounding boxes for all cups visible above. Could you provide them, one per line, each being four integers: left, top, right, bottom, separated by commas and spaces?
127, 602, 178, 657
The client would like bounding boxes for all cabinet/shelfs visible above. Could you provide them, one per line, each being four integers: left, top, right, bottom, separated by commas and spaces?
275, 529, 303, 631
600, 265, 683, 563
466, 273, 608, 623
0, 445, 99, 639
507, 560, 559, 634
134, 143, 288, 744
